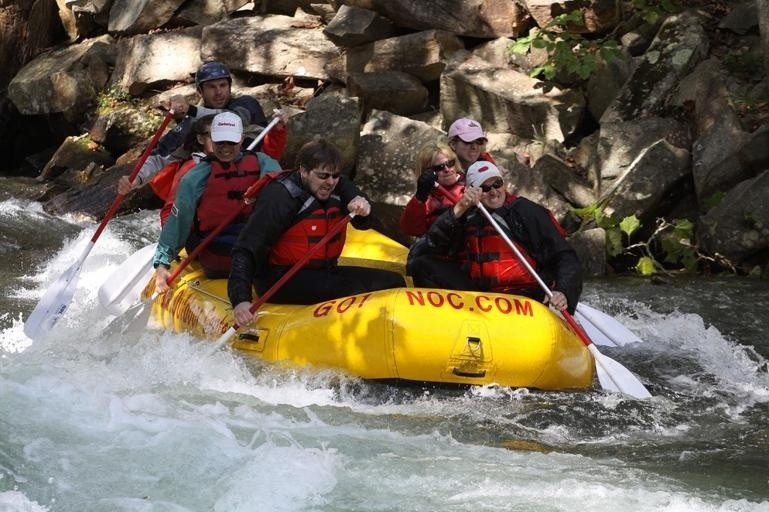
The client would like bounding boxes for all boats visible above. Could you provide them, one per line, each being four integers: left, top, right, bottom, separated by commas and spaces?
142, 221, 594, 391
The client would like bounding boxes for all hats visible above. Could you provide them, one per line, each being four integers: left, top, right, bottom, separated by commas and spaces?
466, 160, 504, 187
448, 118, 488, 143
211, 111, 243, 144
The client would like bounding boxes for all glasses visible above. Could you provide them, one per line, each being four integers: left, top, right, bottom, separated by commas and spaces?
426, 160, 457, 172
310, 168, 340, 179
453, 136, 485, 146
482, 178, 503, 192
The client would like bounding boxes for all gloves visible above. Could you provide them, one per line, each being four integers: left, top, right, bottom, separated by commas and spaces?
415, 173, 435, 201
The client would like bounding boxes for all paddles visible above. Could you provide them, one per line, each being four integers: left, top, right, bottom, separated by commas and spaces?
433, 183, 642, 346
193, 212, 358, 361
478, 203, 654, 402
99, 197, 248, 355
23, 109, 175, 340
98, 117, 281, 317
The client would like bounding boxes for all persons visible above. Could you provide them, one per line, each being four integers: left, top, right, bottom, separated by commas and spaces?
401, 139, 467, 239
225, 137, 408, 327
406, 158, 584, 321
449, 118, 497, 172
130, 116, 290, 232
118, 61, 268, 196
153, 112, 284, 294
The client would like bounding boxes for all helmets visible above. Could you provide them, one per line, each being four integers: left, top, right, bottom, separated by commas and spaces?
194, 61, 232, 90
